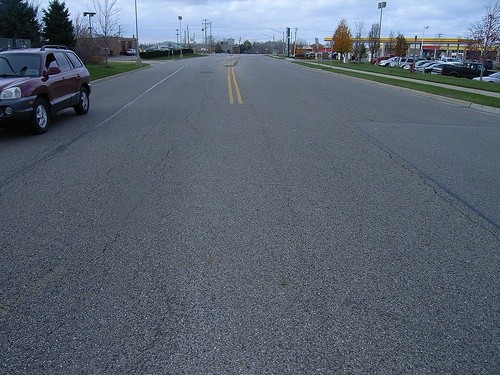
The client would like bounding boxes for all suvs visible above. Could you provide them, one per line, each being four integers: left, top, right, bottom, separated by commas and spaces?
0, 45, 93, 136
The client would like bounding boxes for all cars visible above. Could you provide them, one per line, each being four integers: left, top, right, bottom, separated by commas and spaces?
304, 51, 498, 80
126, 49, 136, 56
473, 71, 500, 85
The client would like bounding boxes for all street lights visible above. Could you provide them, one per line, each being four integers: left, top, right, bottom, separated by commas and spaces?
378, 2, 387, 40
83, 10, 96, 38
